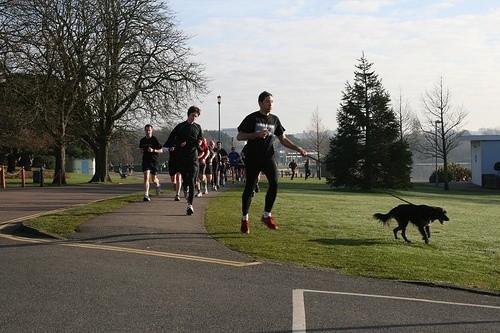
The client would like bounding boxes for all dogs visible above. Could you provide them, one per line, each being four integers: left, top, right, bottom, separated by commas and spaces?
373, 203, 449, 244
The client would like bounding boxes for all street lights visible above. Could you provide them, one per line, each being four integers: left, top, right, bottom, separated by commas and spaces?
216, 94, 222, 143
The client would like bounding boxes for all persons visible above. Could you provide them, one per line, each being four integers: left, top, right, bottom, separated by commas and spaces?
236, 91, 307, 234
289, 160, 298, 180
139, 106, 260, 215
303, 159, 311, 179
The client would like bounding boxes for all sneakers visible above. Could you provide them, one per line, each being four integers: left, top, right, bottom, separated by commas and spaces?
143, 196, 150, 201
187, 204, 194, 216
260, 214, 280, 230
195, 192, 203, 197
174, 195, 181, 201
174, 185, 176, 191
183, 190, 189, 199
203, 191, 208, 194
240, 217, 251, 234
214, 186, 217, 192
155, 184, 160, 189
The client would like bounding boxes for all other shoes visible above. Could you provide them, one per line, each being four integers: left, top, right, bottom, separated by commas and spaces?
251, 191, 255, 198
255, 185, 260, 193
